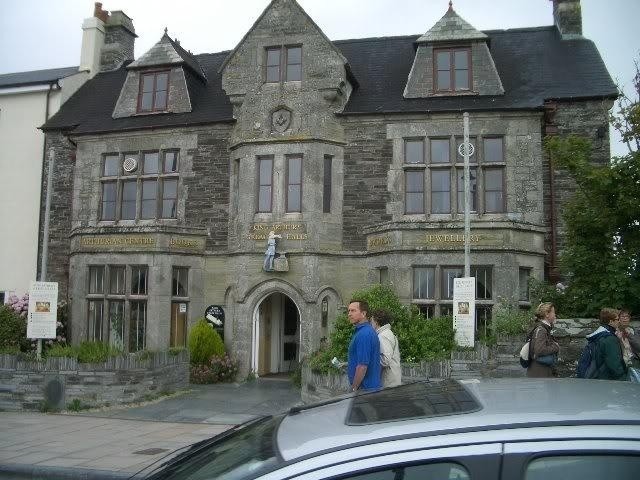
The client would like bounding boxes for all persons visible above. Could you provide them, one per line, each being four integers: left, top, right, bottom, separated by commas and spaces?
337, 299, 381, 394
263, 230, 282, 270
371, 307, 402, 386
615, 309, 640, 383
525, 301, 560, 378
584, 307, 629, 381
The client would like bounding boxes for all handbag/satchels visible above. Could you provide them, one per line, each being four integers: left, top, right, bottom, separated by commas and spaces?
534, 353, 554, 366
520, 338, 531, 369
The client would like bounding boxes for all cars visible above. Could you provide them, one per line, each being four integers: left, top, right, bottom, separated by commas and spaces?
129, 373, 640, 480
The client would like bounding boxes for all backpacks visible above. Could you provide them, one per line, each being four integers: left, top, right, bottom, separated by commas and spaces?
577, 331, 617, 379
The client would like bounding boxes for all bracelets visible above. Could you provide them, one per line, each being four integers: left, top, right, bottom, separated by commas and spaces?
349, 387, 355, 393
353, 383, 360, 388
